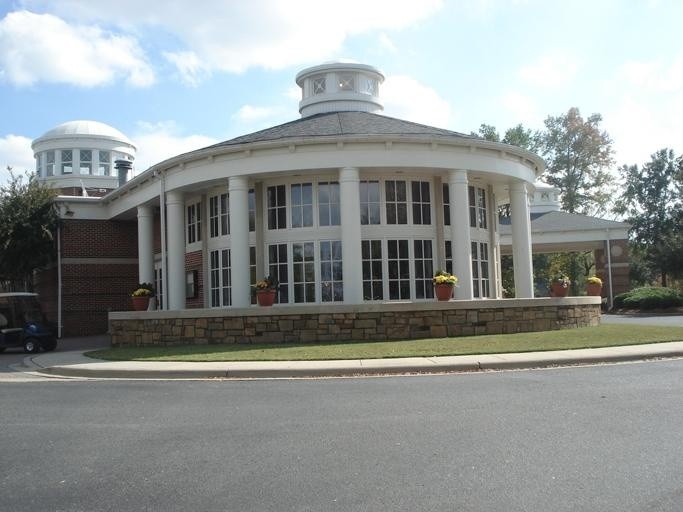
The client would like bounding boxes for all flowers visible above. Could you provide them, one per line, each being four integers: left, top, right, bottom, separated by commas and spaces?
433, 271, 458, 284
552, 271, 569, 289
129, 288, 152, 297
586, 275, 604, 285
251, 278, 277, 289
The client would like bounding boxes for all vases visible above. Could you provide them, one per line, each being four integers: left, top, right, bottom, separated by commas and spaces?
255, 288, 276, 306
550, 280, 566, 296
132, 297, 148, 310
432, 283, 452, 301
589, 281, 603, 295
